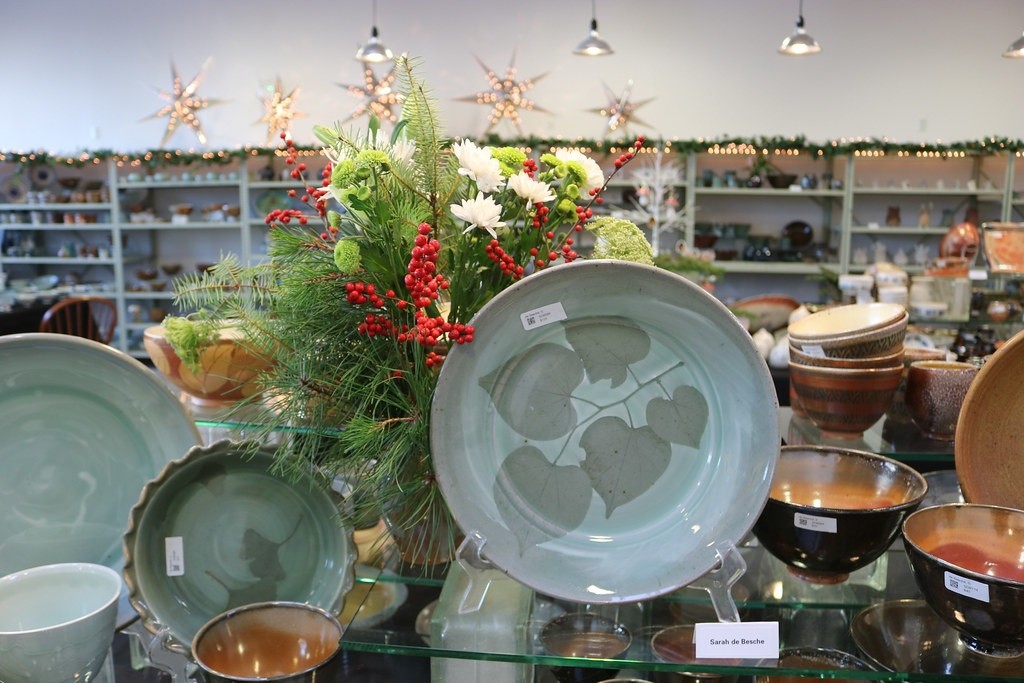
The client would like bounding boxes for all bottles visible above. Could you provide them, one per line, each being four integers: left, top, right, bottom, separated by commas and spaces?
906, 275, 940, 320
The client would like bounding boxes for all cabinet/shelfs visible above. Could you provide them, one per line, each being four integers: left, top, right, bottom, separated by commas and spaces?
0, 136, 1024, 362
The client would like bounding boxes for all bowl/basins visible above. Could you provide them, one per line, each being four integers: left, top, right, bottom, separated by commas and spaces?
539, 612, 631, 683
752, 444, 928, 584
765, 172, 797, 188
903, 502, 1024, 659
192, 601, 345, 683
143, 320, 290, 408
650, 625, 743, 679
133, 268, 158, 278
149, 280, 166, 291
753, 647, 884, 683
0, 562, 124, 683
163, 262, 182, 274
69, 190, 99, 203
16, 289, 58, 307
197, 262, 214, 273
787, 302, 909, 439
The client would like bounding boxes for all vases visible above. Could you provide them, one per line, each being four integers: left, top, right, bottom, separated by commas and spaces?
383, 437, 459, 584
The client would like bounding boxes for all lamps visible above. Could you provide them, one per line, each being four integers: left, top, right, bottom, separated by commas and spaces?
354, 0, 393, 61
574, 0, 614, 56
778, 0, 823, 56
1001, 27, 1024, 58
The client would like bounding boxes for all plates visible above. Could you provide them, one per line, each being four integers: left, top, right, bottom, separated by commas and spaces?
123, 438, 359, 667
724, 295, 800, 334
0, 333, 202, 634
955, 332, 1024, 511
849, 598, 1024, 683
780, 221, 814, 247
429, 260, 784, 607
252, 191, 293, 220
28, 271, 60, 289
340, 560, 406, 630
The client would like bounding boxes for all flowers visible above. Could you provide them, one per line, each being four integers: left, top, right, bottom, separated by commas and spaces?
167, 56, 655, 582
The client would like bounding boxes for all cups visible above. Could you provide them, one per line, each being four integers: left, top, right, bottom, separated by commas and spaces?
703, 168, 716, 184
597, 678, 653, 683
918, 198, 933, 226
884, 348, 976, 440
30, 211, 56, 223
723, 170, 737, 186
887, 197, 902, 226
0, 213, 22, 223
62, 213, 98, 222
